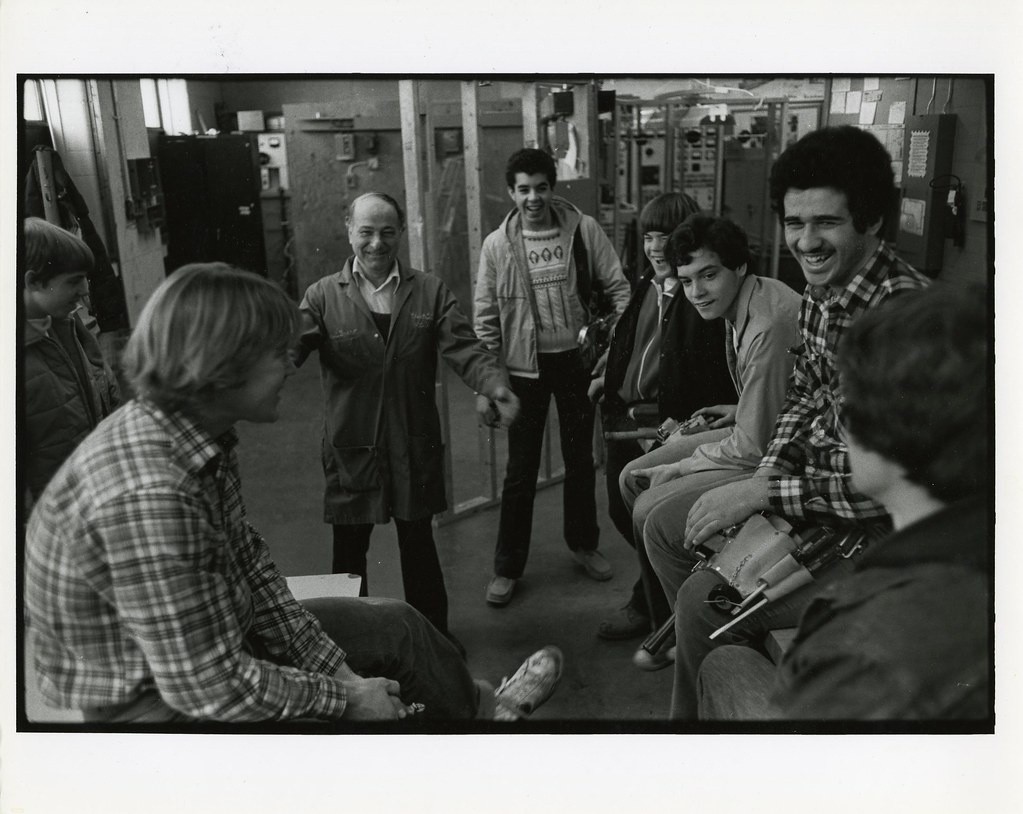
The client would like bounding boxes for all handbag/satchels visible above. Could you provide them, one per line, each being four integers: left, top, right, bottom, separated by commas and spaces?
929, 174, 965, 220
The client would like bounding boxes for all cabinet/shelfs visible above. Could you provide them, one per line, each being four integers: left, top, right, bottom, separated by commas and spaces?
151, 132, 270, 283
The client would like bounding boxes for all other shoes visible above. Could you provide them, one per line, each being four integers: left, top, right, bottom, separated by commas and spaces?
572, 550, 613, 579
598, 602, 660, 640
439, 631, 467, 661
635, 623, 676, 670
486, 574, 516, 603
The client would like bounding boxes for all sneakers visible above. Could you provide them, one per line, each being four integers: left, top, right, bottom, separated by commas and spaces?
492, 645, 566, 721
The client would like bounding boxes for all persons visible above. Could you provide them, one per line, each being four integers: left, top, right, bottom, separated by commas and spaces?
598, 121, 991, 722
280, 188, 522, 672
21, 214, 127, 507
470, 146, 633, 608
21, 260, 567, 726
